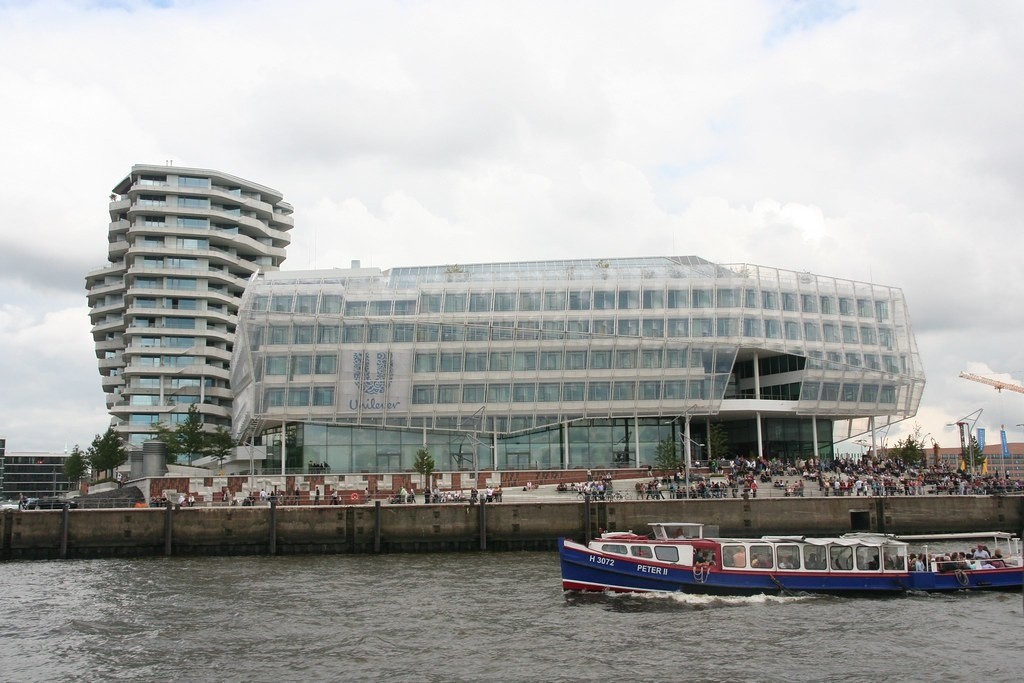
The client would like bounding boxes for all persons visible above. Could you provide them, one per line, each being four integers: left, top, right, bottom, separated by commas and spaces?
117, 474, 128, 488
177, 493, 194, 507
657, 527, 686, 539
557, 467, 624, 500
314, 487, 320, 505
693, 543, 1012, 571
150, 493, 167, 507
532, 483, 539, 489
636, 455, 1024, 502
18, 493, 29, 510
390, 485, 502, 504
308, 461, 330, 474
293, 487, 300, 505
222, 489, 284, 506
329, 488, 341, 505
365, 487, 369, 504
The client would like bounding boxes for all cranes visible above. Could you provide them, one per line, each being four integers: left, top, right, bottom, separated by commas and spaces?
958, 371, 1024, 395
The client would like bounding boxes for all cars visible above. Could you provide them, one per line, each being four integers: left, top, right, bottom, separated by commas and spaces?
25, 499, 78, 510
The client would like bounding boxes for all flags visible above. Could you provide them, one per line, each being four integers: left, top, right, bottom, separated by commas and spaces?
1001, 431, 1010, 458
977, 428, 985, 452
959, 423, 966, 460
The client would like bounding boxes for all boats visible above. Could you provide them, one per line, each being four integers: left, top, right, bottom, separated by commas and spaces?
840, 532, 1024, 590
558, 523, 910, 597
